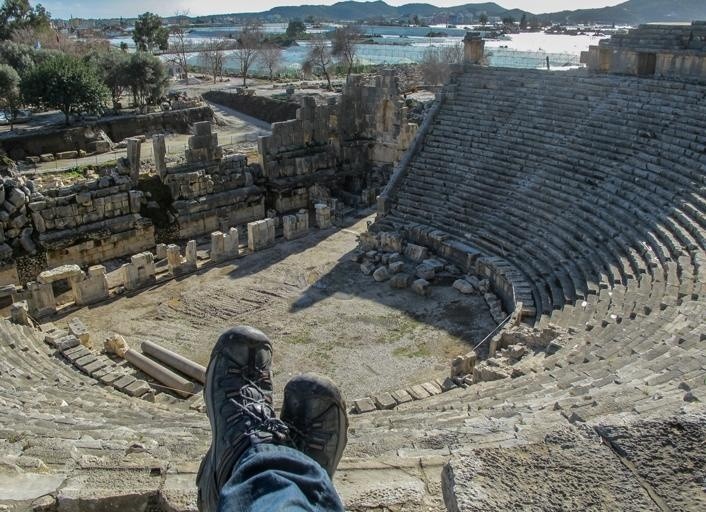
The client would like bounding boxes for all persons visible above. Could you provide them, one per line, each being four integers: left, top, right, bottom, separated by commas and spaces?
196, 324, 349, 512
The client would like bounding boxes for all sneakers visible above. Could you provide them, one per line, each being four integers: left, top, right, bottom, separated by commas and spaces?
281, 374, 348, 478
196, 326, 290, 512
